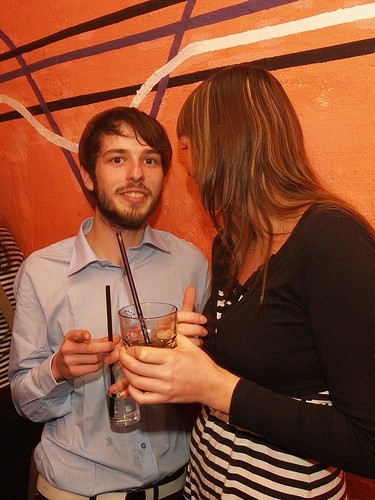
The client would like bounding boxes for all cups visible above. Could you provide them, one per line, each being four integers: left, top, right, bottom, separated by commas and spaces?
101, 361, 140, 427
118, 302, 177, 349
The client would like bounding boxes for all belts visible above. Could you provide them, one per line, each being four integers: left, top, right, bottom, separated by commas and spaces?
35, 468, 185, 500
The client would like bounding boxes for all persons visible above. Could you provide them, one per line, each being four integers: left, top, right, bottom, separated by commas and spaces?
118, 64, 375, 500
0, 225, 34, 500
8, 105, 211, 500
